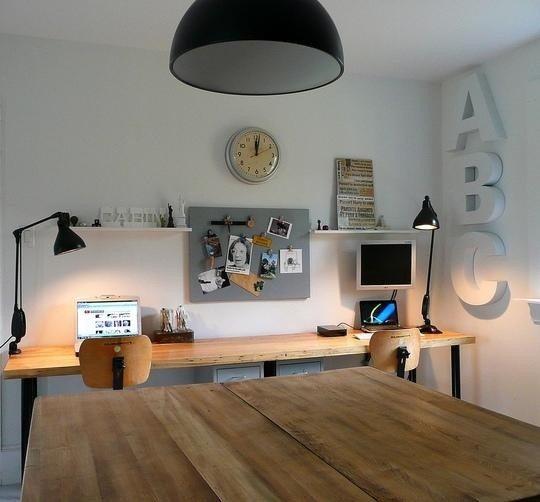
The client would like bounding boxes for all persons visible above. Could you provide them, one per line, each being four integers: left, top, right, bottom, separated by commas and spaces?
167, 202, 174, 220
227, 237, 252, 271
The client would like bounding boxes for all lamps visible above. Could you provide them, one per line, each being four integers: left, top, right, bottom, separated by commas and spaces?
8, 211, 87, 356
168, 0, 346, 97
412, 193, 443, 334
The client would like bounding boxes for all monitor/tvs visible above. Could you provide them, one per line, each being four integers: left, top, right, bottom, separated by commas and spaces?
355, 239, 417, 290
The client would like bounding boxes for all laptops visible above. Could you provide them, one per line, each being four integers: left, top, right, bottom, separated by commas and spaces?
359, 300, 402, 333
73, 294, 142, 357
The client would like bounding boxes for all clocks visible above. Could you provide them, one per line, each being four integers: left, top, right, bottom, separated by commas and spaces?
225, 126, 282, 186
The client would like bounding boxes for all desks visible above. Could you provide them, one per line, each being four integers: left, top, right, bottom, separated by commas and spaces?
2, 325, 476, 485
21, 383, 377, 502
221, 367, 540, 501
